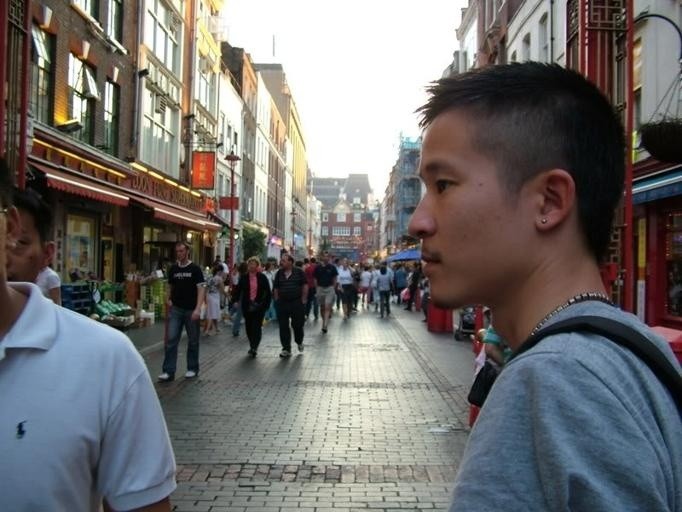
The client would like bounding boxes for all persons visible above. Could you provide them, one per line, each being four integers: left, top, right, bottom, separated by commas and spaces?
202, 251, 431, 338
1, 159, 178, 511
470, 302, 515, 430
231, 256, 271, 358
5, 180, 56, 285
406, 57, 682, 511
273, 253, 311, 358
36, 258, 65, 305
157, 241, 205, 382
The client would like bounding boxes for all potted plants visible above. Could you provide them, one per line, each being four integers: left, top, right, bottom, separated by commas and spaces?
637, 117, 681, 164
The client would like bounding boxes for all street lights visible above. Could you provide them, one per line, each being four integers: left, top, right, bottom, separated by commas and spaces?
306, 225, 314, 261
288, 205, 299, 256
225, 147, 242, 275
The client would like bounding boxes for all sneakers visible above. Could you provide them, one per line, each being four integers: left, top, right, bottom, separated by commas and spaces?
248, 347, 257, 356
157, 372, 175, 380
322, 326, 327, 333
336, 302, 390, 319
279, 350, 292, 357
403, 307, 412, 310
298, 342, 305, 351
202, 329, 240, 338
184, 368, 199, 378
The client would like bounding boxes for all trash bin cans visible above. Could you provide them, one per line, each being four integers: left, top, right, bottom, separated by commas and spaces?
427, 296, 452, 333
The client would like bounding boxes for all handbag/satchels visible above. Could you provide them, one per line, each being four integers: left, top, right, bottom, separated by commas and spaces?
468, 360, 499, 408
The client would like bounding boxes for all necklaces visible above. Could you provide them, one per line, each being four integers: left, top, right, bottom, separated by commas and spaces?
524, 290, 618, 339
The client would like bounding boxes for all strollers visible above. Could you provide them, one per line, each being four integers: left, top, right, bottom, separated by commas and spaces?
456, 304, 482, 342
